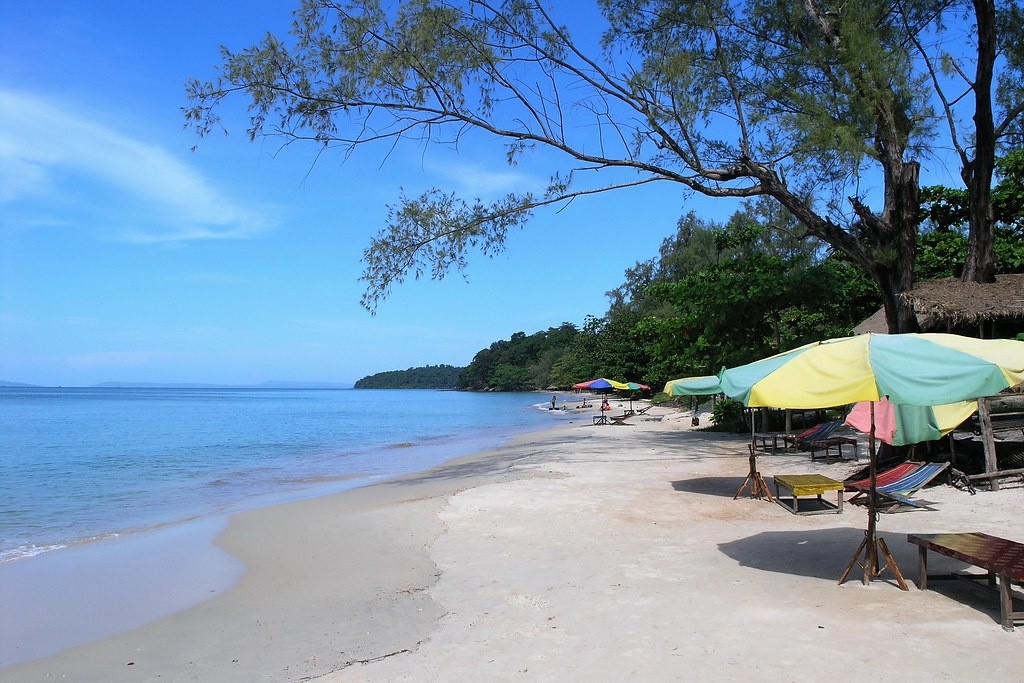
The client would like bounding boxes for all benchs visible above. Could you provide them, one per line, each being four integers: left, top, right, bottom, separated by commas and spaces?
908, 532, 1024, 633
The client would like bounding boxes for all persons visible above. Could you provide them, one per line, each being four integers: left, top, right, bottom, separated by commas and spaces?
550, 395, 556, 410
601, 393, 609, 411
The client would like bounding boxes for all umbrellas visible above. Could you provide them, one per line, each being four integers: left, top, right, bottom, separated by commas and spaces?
615, 381, 653, 414
573, 376, 616, 418
661, 376, 763, 460
840, 392, 980, 447
714, 327, 1024, 590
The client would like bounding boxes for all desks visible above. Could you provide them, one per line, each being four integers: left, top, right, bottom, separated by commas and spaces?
754, 433, 787, 454
811, 436, 858, 464
592, 416, 606, 425
774, 473, 844, 515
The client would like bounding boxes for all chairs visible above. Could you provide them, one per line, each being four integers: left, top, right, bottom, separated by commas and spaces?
610, 412, 631, 426
796, 422, 842, 451
876, 461, 950, 514
780, 422, 823, 450
849, 460, 926, 505
